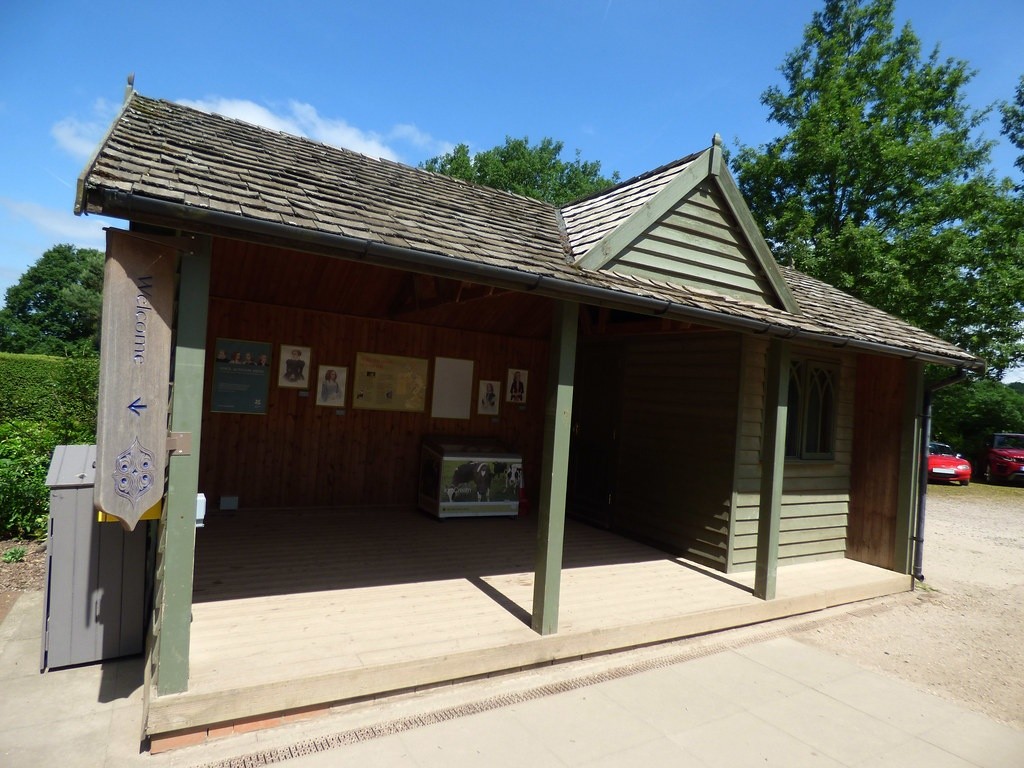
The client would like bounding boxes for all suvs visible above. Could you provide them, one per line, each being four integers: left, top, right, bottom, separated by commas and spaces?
978, 432, 1024, 486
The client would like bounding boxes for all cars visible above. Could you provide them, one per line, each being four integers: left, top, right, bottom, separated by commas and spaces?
928, 442, 972, 485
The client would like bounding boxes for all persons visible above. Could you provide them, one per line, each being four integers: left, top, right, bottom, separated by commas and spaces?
284, 350, 305, 383
482, 383, 496, 408
319, 369, 343, 403
218, 348, 269, 368
510, 371, 525, 403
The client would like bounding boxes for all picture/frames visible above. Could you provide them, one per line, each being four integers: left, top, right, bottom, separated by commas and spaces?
209, 337, 273, 415
430, 356, 477, 420
314, 364, 349, 408
504, 366, 530, 404
477, 377, 502, 417
276, 343, 312, 390
350, 350, 429, 413
